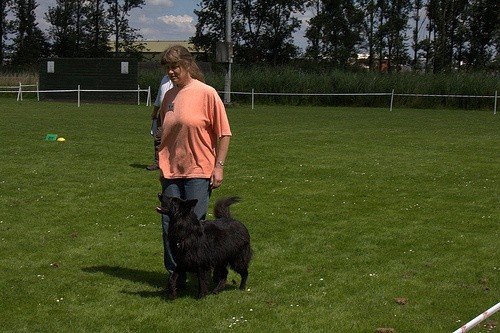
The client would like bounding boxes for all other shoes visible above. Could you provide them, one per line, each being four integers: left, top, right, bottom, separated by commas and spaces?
209, 270, 229, 293
168, 272, 187, 289
146, 164, 159, 170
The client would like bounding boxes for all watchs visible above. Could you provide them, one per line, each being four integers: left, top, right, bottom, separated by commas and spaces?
216, 160, 224, 166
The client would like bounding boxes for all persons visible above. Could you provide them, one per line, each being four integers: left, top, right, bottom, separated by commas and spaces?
154, 44, 233, 289
145, 71, 175, 172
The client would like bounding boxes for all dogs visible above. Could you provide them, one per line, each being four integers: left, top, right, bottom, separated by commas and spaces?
156, 192, 254, 300
